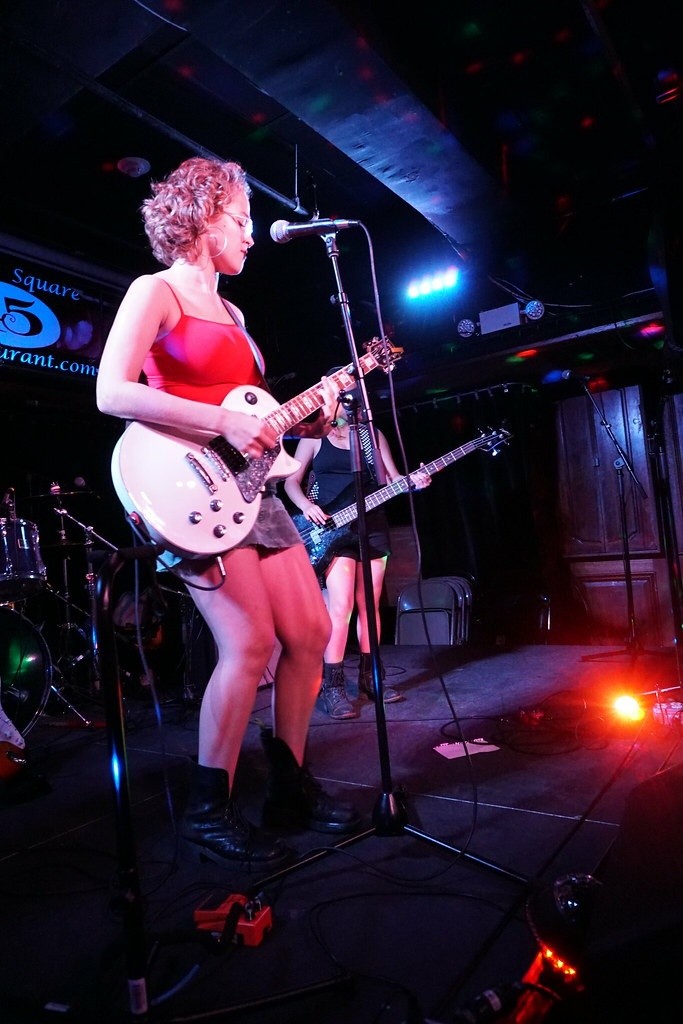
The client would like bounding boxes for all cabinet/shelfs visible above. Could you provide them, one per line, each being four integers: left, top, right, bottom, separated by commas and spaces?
550, 386, 683, 646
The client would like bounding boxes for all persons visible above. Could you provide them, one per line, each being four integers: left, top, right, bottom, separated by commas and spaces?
285, 365, 433, 719
94, 154, 363, 874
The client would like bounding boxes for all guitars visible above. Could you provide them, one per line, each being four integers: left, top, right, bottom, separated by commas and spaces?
110, 334, 404, 561
291, 424, 515, 577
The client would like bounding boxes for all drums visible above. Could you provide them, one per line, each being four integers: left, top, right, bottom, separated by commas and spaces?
0, 516, 48, 604
0, 604, 55, 738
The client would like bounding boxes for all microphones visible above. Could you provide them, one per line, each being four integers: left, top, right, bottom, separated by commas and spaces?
15, 690, 30, 704
270, 219, 361, 244
75, 477, 101, 501
0, 487, 14, 518
562, 370, 591, 381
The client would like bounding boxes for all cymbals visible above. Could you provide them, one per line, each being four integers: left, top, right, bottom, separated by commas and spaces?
41, 541, 94, 550
22, 490, 100, 504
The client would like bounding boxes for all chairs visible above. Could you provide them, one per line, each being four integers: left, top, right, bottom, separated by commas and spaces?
396, 576, 473, 647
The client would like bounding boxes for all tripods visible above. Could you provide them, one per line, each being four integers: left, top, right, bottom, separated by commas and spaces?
45, 494, 205, 715
579, 380, 676, 680
244, 234, 557, 902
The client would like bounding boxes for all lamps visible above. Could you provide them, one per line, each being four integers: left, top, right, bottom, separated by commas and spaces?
459, 302, 544, 338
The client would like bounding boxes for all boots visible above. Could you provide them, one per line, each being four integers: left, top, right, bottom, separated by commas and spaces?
259, 728, 361, 831
358, 652, 403, 703
320, 661, 356, 719
179, 761, 300, 869
